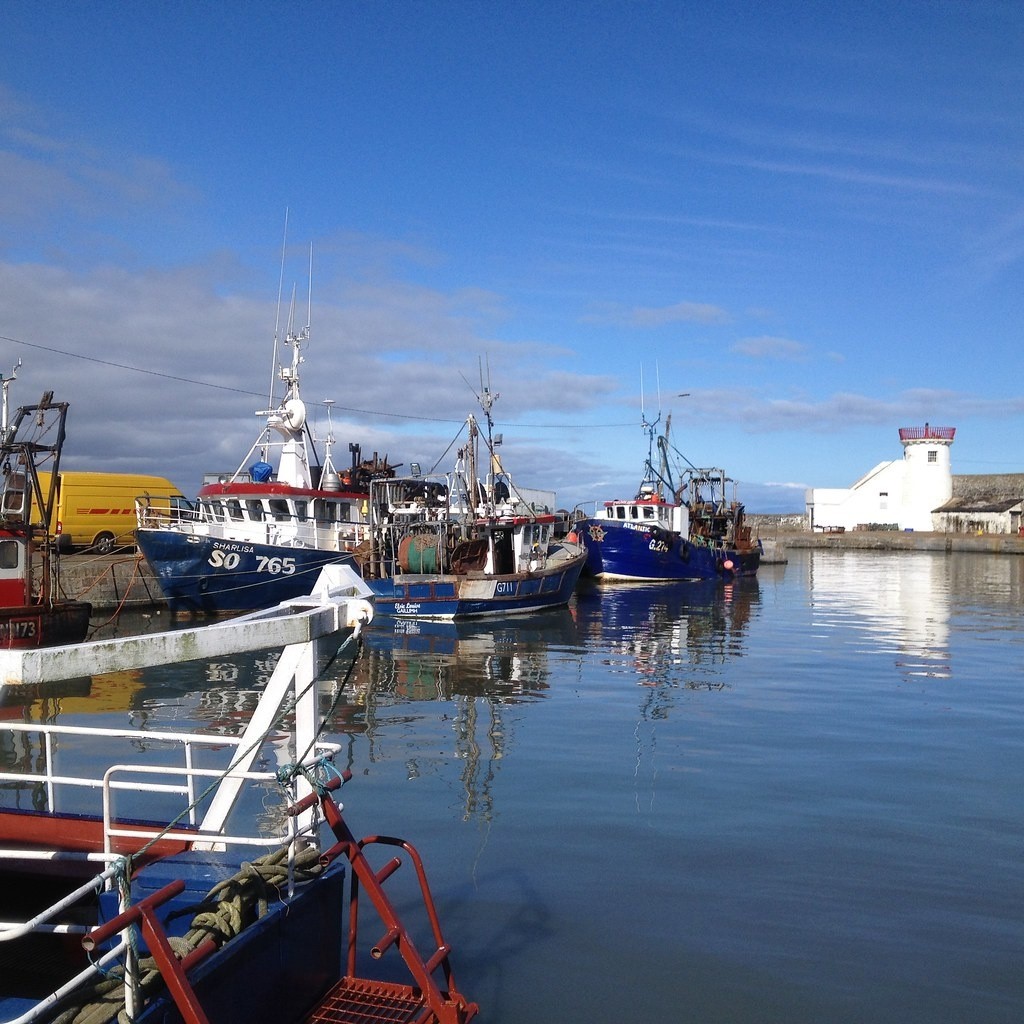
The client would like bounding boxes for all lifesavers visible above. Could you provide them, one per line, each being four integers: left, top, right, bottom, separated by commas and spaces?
663, 532, 672, 542
740, 560, 747, 567
344, 527, 364, 551
679, 544, 690, 564
650, 525, 660, 538
715, 557, 725, 574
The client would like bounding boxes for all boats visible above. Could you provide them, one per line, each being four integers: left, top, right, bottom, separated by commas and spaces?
573, 354, 763, 588
1, 387, 91, 650
131, 205, 587, 626
0, 560, 480, 1024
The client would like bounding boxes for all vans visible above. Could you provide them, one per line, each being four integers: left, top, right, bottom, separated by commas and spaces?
1, 469, 197, 553
